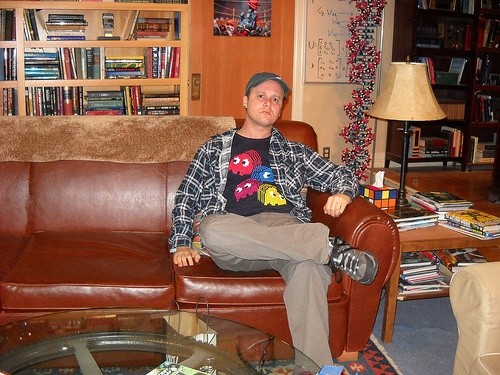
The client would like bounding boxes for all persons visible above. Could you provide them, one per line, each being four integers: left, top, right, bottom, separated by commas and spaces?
167, 72, 379, 375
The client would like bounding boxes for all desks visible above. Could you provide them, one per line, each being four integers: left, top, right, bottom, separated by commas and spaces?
366, 167, 500, 342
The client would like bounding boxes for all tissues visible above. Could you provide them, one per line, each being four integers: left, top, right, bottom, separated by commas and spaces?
360, 170, 398, 210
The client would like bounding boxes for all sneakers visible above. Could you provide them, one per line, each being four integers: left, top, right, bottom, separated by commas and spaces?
330, 236, 378, 285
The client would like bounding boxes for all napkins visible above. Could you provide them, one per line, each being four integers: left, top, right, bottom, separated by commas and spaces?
373, 171, 385, 188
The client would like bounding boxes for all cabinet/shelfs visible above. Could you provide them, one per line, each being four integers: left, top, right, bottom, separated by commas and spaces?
0, 0, 192, 116
387, 0, 500, 171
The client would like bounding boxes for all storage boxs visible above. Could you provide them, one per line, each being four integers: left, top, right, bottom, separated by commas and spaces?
364, 184, 397, 210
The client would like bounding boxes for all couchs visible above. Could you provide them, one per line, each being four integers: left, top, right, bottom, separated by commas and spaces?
449, 262, 500, 375
0, 116, 400, 365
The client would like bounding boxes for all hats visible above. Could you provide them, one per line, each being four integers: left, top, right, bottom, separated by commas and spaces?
246, 72, 290, 94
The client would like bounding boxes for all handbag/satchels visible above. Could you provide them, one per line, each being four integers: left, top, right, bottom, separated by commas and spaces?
162, 296, 217, 374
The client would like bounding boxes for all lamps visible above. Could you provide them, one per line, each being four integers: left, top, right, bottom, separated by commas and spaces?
370, 56, 446, 208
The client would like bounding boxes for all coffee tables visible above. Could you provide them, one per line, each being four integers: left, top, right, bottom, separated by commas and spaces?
0, 306, 321, 375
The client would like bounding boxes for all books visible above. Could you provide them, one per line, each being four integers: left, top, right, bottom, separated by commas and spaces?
0, 48, 17, 81
480, 0, 491, 16
105, 47, 180, 79
0, 8, 16, 41
24, 47, 100, 80
413, 23, 473, 50
414, 56, 466, 85
122, 10, 182, 40
408, 126, 464, 158
470, 136, 496, 164
478, 17, 500, 48
418, 0, 475, 14
145, 361, 212, 375
0, 87, 18, 116
475, 55, 500, 86
23, 8, 88, 41
397, 247, 488, 295
97, 36, 120, 40
381, 191, 500, 240
477, 94, 494, 122
25, 85, 180, 116
